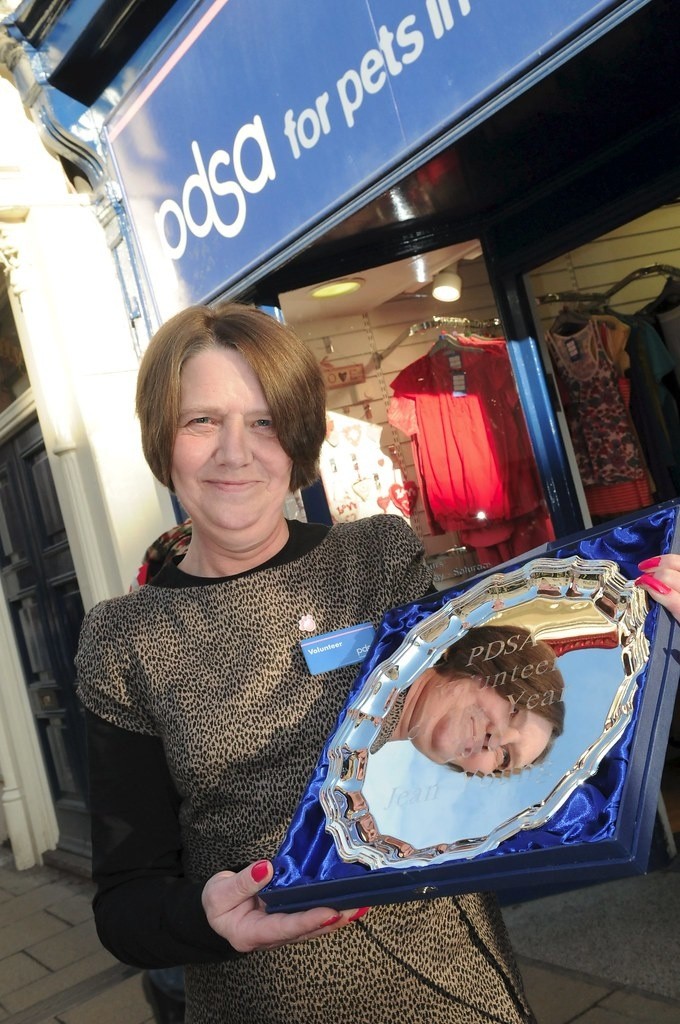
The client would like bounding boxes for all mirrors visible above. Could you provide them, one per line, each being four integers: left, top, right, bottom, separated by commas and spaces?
259, 497, 680, 915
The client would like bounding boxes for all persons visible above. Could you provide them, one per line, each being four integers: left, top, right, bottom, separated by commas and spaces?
370, 625, 566, 779
72, 300, 680, 1024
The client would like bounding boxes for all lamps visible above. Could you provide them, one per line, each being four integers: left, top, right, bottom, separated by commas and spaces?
431, 263, 462, 302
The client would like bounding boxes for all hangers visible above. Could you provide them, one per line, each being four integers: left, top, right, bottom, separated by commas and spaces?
424, 289, 610, 360
